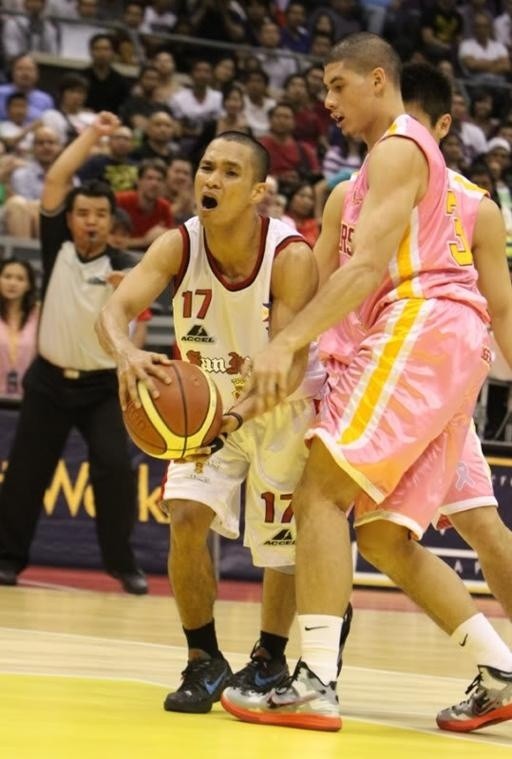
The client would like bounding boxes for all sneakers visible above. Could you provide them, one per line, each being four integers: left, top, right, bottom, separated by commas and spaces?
230, 647, 291, 691
222, 656, 342, 730
164, 650, 233, 712
436, 665, 511, 730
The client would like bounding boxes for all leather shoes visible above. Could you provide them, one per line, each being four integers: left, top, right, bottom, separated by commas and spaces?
113, 564, 146, 592
0, 565, 18, 586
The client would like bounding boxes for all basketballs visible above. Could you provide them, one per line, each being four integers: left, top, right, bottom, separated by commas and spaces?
121, 361, 223, 459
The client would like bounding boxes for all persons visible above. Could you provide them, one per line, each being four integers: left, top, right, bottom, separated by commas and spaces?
0, 3, 511, 439
95, 131, 319, 713
0, 110, 148, 595
220, 36, 512, 730
313, 64, 512, 675
